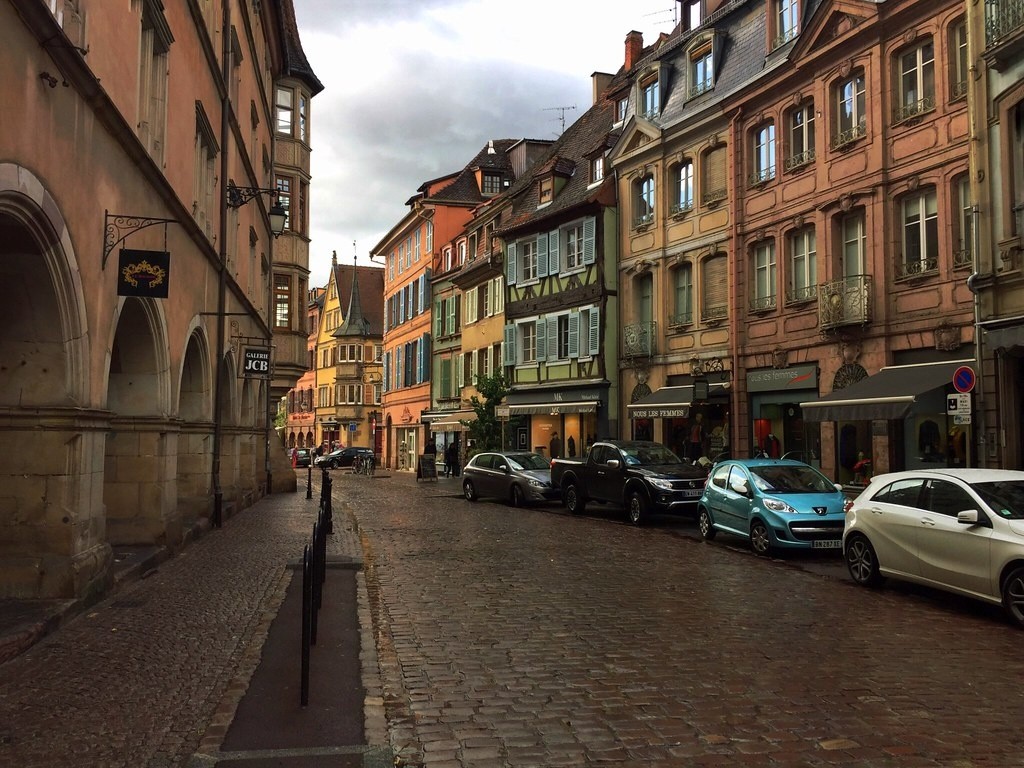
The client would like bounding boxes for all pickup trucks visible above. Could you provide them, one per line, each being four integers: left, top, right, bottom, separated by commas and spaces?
550, 440, 707, 525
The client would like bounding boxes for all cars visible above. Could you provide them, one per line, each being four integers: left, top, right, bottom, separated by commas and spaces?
696, 460, 849, 554
841, 468, 1024, 626
462, 452, 551, 505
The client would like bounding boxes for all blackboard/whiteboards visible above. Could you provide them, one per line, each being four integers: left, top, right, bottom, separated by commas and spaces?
419, 454, 438, 478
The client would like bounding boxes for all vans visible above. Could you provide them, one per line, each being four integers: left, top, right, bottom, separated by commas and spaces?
314, 447, 374, 469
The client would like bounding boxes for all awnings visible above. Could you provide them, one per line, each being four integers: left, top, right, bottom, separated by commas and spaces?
431, 412, 478, 432
626, 382, 731, 419
506, 381, 610, 415
800, 357, 974, 421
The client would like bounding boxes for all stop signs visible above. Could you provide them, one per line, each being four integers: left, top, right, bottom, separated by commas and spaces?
372, 419, 376, 428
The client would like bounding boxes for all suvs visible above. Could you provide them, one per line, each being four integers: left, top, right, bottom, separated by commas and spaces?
287, 448, 311, 465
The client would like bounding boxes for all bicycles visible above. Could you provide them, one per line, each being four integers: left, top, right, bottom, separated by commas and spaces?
352, 456, 364, 475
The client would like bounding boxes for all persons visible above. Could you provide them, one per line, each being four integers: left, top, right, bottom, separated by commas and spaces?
850, 451, 873, 486
424, 438, 436, 458
568, 436, 575, 457
550, 432, 562, 461
445, 443, 458, 478
682, 413, 706, 463
721, 412, 729, 454
292, 448, 299, 468
316, 441, 343, 457
764, 434, 780, 459
946, 426, 966, 468
918, 419, 940, 455
